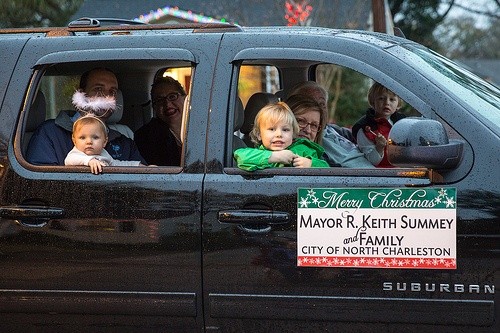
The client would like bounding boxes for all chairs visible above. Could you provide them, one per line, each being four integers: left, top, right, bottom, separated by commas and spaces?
106, 91, 134, 140
24, 91, 46, 150
232, 95, 248, 168
240, 93, 280, 147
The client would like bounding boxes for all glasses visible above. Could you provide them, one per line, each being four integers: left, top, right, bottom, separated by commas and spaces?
152, 92, 182, 105
296, 119, 322, 132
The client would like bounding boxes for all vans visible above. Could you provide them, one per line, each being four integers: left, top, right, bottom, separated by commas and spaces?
0, 16, 496, 333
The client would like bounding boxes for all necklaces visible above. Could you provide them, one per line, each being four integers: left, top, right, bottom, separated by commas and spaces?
168, 127, 182, 144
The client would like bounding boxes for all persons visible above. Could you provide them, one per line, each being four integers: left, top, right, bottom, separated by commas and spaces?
133, 76, 187, 166
283, 94, 328, 159
64, 115, 156, 174
26, 67, 148, 166
233, 101, 330, 171
352, 81, 408, 168
287, 81, 376, 167
